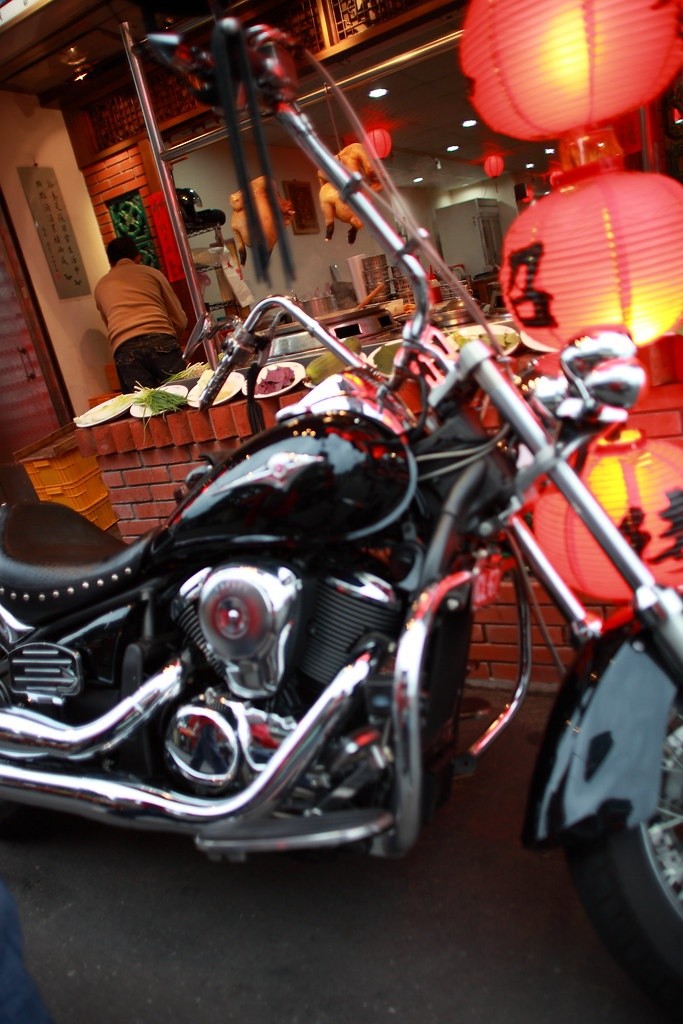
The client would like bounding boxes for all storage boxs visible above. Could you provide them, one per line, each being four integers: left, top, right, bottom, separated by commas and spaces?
24, 446, 119, 531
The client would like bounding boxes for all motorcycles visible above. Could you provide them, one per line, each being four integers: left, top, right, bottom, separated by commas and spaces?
0, 11, 683, 1022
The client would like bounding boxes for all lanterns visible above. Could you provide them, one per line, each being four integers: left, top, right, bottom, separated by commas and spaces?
550, 171, 565, 185
483, 155, 505, 179
364, 127, 392, 160
531, 426, 683, 603
498, 165, 683, 350
459, 0, 683, 166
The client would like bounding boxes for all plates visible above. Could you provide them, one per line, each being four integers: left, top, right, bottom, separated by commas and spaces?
366, 337, 402, 379
241, 362, 306, 398
130, 385, 188, 417
76, 393, 134, 427
302, 351, 368, 388
186, 371, 245, 408
445, 324, 520, 357
520, 331, 563, 352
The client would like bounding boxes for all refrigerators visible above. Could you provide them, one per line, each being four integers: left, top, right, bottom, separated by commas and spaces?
435, 198, 505, 280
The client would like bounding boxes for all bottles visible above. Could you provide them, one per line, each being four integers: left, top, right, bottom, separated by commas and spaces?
429, 275, 443, 304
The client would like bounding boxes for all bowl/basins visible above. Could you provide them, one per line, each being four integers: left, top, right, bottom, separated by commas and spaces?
382, 298, 405, 317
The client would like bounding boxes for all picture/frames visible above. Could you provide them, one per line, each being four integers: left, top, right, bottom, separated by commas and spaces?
283, 179, 321, 235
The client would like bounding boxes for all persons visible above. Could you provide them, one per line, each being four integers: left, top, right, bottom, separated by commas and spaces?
93, 235, 188, 396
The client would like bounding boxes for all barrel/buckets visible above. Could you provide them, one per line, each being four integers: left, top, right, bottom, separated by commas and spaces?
301, 295, 334, 317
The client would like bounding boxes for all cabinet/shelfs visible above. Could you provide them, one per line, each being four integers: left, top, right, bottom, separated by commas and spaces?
185, 223, 250, 323
